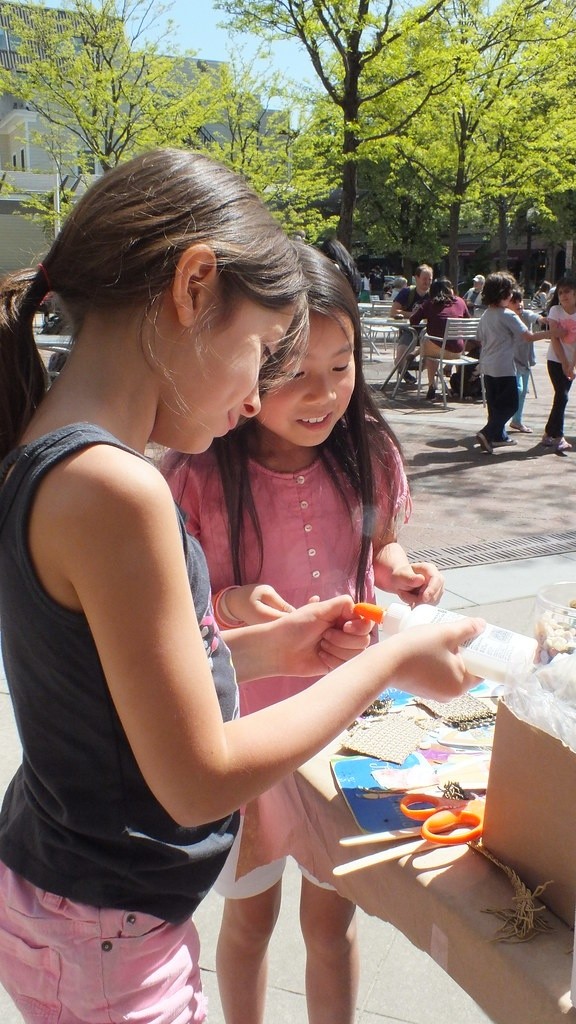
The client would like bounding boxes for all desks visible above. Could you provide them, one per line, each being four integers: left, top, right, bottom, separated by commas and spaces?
258, 702, 576, 1024
357, 302, 393, 342
33, 333, 76, 391
360, 317, 454, 399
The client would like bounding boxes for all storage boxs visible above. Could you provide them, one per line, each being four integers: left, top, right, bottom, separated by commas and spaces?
482, 687, 576, 930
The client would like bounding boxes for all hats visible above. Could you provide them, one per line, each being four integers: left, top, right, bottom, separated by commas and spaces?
472, 275, 486, 284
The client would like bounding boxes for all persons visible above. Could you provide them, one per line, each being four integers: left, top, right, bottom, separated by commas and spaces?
156, 231, 442, 1024
475, 271, 568, 454
542, 279, 576, 451
409, 277, 471, 402
392, 277, 407, 300
505, 288, 549, 433
391, 264, 433, 386
0, 150, 486, 1024
532, 281, 553, 331
461, 274, 490, 351
361, 266, 385, 299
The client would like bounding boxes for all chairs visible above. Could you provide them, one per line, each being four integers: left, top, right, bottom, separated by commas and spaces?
362, 296, 549, 410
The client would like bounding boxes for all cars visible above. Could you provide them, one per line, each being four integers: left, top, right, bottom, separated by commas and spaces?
384, 276, 395, 284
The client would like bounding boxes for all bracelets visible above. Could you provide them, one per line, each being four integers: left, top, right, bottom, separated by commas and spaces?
213, 585, 246, 628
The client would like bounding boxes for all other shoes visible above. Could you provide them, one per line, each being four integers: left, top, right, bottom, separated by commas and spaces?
476, 432, 493, 454
553, 439, 572, 452
405, 354, 415, 361
509, 422, 532, 434
491, 438, 517, 447
542, 435, 554, 445
426, 387, 435, 399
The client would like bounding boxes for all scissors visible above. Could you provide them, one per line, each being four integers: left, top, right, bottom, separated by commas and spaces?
400, 793, 485, 844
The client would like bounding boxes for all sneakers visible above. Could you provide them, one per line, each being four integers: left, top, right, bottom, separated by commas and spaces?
404, 374, 416, 384
